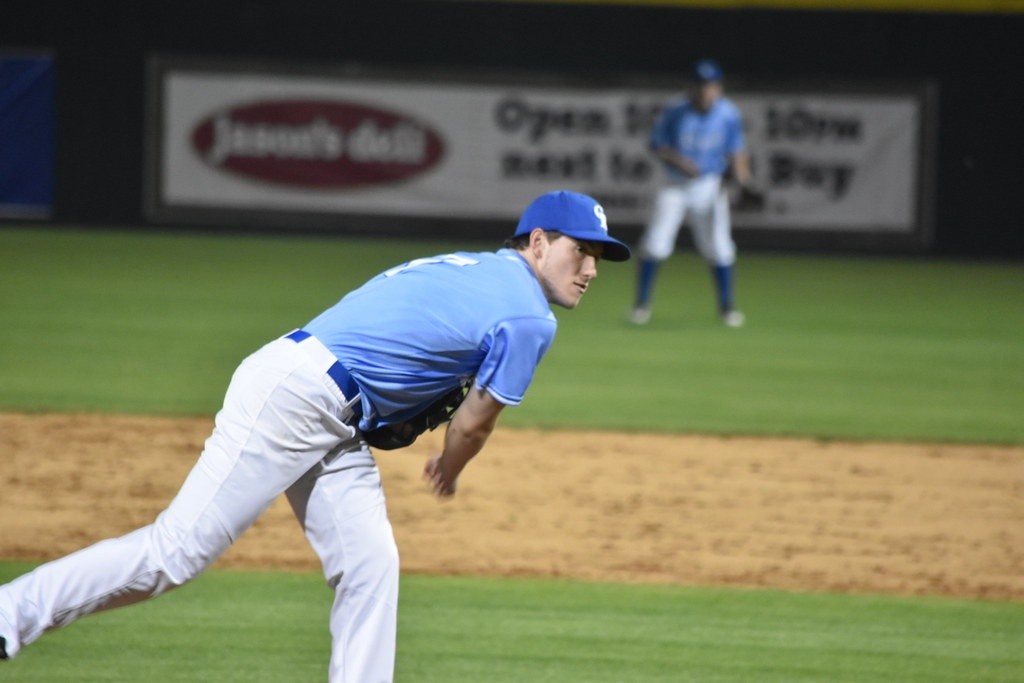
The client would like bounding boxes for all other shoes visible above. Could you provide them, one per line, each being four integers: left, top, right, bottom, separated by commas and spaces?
629, 305, 651, 327
724, 310, 746, 328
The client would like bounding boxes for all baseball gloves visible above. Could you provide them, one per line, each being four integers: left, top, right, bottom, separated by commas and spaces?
736, 179, 767, 207
363, 378, 473, 452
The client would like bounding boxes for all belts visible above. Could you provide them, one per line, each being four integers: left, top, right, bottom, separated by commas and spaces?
285, 330, 362, 430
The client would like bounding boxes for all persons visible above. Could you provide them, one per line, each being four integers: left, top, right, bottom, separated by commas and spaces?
630, 57, 762, 326
1, 192, 632, 683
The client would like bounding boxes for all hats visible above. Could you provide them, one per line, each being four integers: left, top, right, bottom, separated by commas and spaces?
514, 191, 632, 262
684, 63, 718, 83
